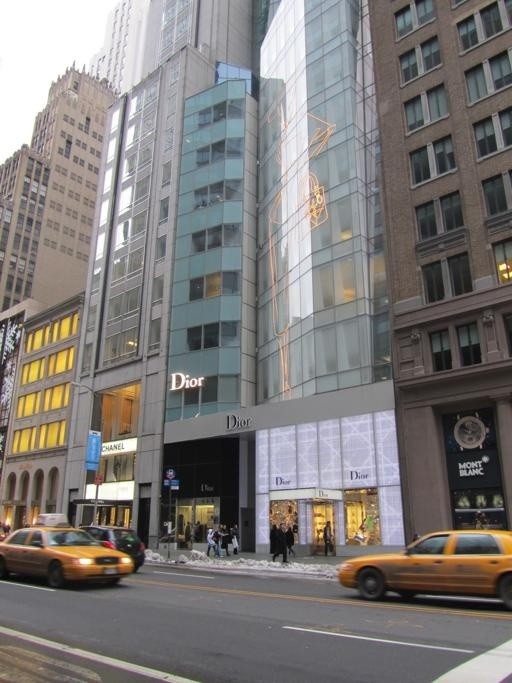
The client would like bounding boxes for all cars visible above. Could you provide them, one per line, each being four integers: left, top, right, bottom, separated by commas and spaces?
338, 529, 512, 610
0, 525, 146, 588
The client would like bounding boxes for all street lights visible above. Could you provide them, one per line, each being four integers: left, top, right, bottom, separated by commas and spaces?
71, 381, 105, 525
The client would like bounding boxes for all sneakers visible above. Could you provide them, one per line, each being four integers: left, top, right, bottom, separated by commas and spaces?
214, 554, 225, 558
272, 556, 288, 562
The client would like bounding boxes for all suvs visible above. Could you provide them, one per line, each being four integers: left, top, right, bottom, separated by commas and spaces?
36, 513, 70, 529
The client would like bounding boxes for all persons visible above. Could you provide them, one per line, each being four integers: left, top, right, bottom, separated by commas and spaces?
269, 522, 295, 563
183, 517, 240, 558
323, 520, 335, 555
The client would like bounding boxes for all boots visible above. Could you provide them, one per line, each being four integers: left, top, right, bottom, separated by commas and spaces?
234, 547, 238, 555
